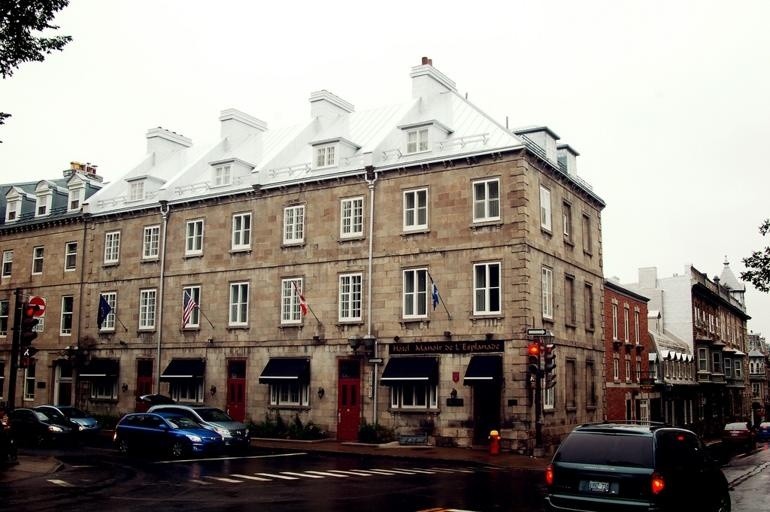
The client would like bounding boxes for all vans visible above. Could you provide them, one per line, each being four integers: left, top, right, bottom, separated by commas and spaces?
138, 393, 251, 452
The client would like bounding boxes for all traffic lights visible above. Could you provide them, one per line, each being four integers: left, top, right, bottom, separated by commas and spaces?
546, 370, 557, 389
527, 372, 540, 390
20, 302, 42, 347
545, 343, 556, 373
527, 343, 541, 375
21, 344, 32, 368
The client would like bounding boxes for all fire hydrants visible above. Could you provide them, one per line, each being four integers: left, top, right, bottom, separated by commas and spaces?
488, 429, 502, 455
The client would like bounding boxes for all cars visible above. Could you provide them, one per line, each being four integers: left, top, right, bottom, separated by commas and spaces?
720, 420, 757, 452
34, 402, 103, 439
13, 407, 80, 447
111, 412, 223, 460
757, 421, 770, 441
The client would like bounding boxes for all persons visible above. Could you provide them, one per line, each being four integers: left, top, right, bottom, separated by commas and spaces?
1, 407, 18, 465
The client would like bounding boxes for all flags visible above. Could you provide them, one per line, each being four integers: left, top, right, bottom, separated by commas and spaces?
299, 290, 307, 316
96, 294, 111, 329
183, 290, 199, 328
432, 281, 439, 311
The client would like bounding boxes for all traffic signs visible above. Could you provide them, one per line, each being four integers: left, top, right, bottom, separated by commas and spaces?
30, 316, 45, 333
527, 328, 548, 336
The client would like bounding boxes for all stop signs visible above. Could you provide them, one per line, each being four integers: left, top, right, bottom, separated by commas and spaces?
28, 295, 47, 318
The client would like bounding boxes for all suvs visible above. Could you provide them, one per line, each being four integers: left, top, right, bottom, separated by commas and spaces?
545, 418, 732, 512
